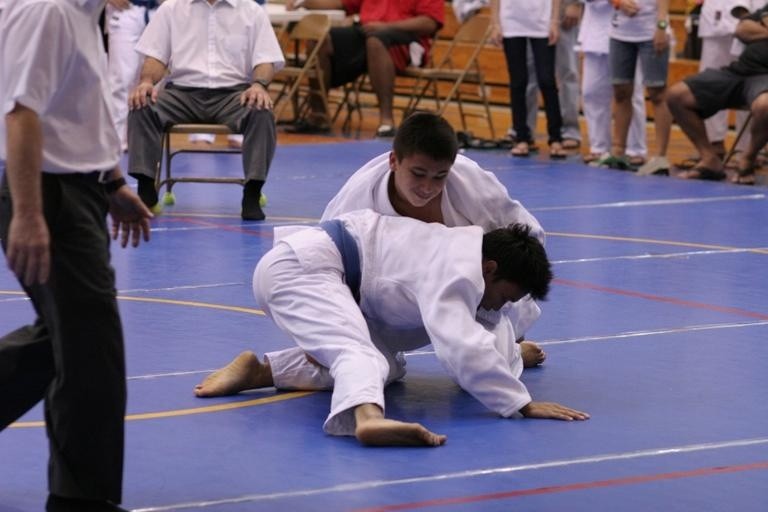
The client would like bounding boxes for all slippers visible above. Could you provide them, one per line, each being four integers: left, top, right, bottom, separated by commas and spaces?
511, 137, 757, 186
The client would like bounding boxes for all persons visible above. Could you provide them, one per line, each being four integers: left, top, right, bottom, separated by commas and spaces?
317, 111, 548, 368
104, 1, 166, 151
273, 1, 768, 183
0, 1, 155, 512
126, 0, 287, 219
194, 207, 590, 445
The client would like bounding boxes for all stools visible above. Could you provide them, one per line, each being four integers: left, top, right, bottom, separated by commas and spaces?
676, 94, 762, 175
149, 123, 269, 220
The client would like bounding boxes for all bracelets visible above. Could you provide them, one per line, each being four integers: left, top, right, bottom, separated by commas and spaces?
104, 176, 125, 196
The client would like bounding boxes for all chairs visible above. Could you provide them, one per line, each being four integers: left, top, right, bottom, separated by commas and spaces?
271, 14, 494, 140
